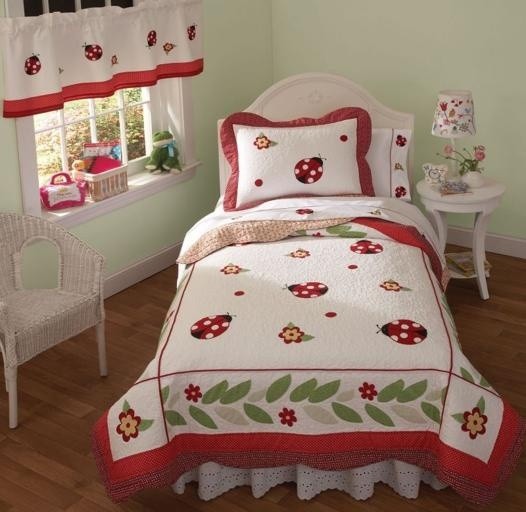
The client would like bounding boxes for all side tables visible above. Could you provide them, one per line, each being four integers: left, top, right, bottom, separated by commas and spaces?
416, 179, 506, 300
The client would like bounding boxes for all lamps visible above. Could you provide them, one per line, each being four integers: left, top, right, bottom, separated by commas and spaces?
432, 90, 477, 181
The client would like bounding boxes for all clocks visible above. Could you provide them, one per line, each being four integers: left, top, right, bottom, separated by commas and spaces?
422, 163, 448, 187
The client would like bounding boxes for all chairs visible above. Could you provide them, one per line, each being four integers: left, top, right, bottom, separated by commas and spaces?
0, 212, 109, 428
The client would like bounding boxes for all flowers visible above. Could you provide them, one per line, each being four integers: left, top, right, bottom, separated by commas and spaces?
436, 144, 486, 177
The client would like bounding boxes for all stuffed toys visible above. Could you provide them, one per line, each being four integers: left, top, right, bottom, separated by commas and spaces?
145, 130, 182, 175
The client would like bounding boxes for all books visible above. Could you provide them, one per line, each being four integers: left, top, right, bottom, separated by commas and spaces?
445, 256, 475, 277
446, 251, 492, 271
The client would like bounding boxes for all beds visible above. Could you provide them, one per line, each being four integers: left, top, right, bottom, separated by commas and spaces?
88, 72, 526, 507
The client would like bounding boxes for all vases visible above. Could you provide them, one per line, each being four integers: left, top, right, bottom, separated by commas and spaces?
463, 171, 486, 187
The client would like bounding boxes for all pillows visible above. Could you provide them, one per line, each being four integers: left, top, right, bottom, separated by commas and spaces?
367, 128, 412, 202
220, 107, 376, 212
